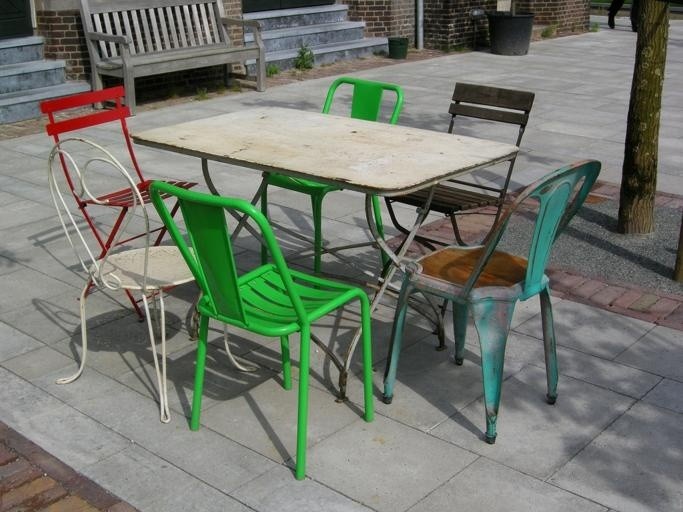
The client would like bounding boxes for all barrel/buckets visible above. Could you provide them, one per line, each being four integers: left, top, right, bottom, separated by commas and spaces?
485, 10, 536, 56
389, 37, 408, 59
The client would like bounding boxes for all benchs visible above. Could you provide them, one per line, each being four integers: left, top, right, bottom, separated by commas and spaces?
80, 0, 266, 116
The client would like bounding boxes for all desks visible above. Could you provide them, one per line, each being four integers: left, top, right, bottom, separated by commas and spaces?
130, 106, 518, 403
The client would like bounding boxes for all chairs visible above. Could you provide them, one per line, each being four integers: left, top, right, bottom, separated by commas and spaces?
384, 83, 535, 334
149, 180, 375, 481
261, 77, 404, 273
48, 137, 257, 423
40, 86, 198, 320
382, 159, 601, 444
